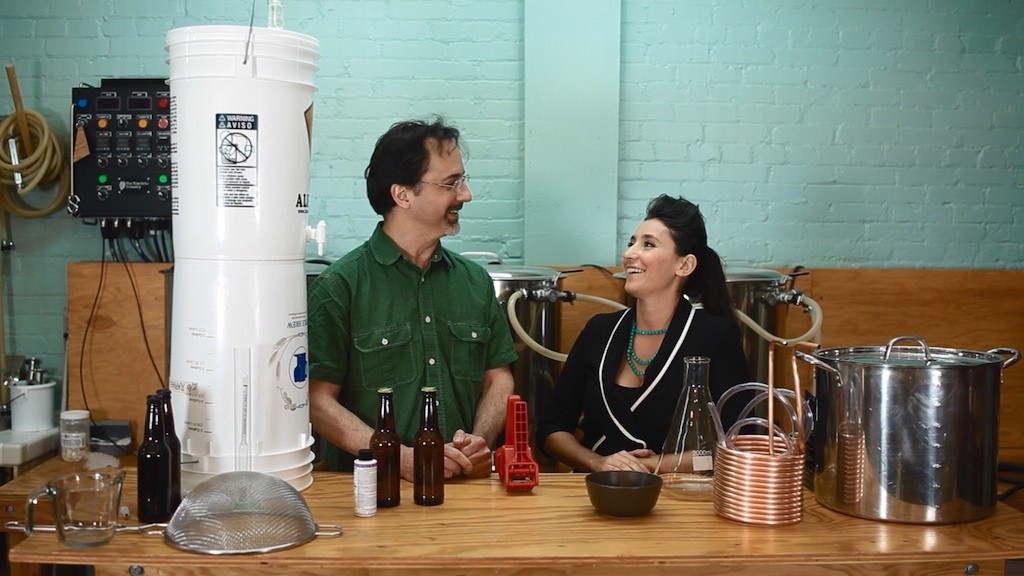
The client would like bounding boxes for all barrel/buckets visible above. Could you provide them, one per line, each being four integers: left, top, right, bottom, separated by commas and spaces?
6, 379, 56, 431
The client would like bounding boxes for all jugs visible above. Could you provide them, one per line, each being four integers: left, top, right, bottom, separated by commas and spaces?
25, 471, 127, 549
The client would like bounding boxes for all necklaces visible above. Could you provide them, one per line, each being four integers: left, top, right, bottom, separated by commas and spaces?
626, 320, 668, 375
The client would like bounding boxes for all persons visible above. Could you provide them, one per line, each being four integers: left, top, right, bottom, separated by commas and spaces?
307, 111, 520, 484
535, 193, 756, 474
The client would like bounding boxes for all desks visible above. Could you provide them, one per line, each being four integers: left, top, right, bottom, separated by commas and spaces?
13, 470, 1024, 575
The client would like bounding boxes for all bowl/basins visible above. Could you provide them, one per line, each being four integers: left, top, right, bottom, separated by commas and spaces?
585, 470, 663, 516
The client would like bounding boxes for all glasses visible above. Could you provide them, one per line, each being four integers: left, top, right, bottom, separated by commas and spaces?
418, 174, 470, 193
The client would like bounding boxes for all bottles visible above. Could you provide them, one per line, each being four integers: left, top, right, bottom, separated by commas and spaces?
414, 387, 444, 506
59, 410, 91, 462
138, 395, 171, 524
354, 449, 378, 517
370, 388, 400, 508
154, 388, 182, 520
653, 356, 717, 502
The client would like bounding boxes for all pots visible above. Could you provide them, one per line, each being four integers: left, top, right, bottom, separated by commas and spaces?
795, 336, 1021, 523
